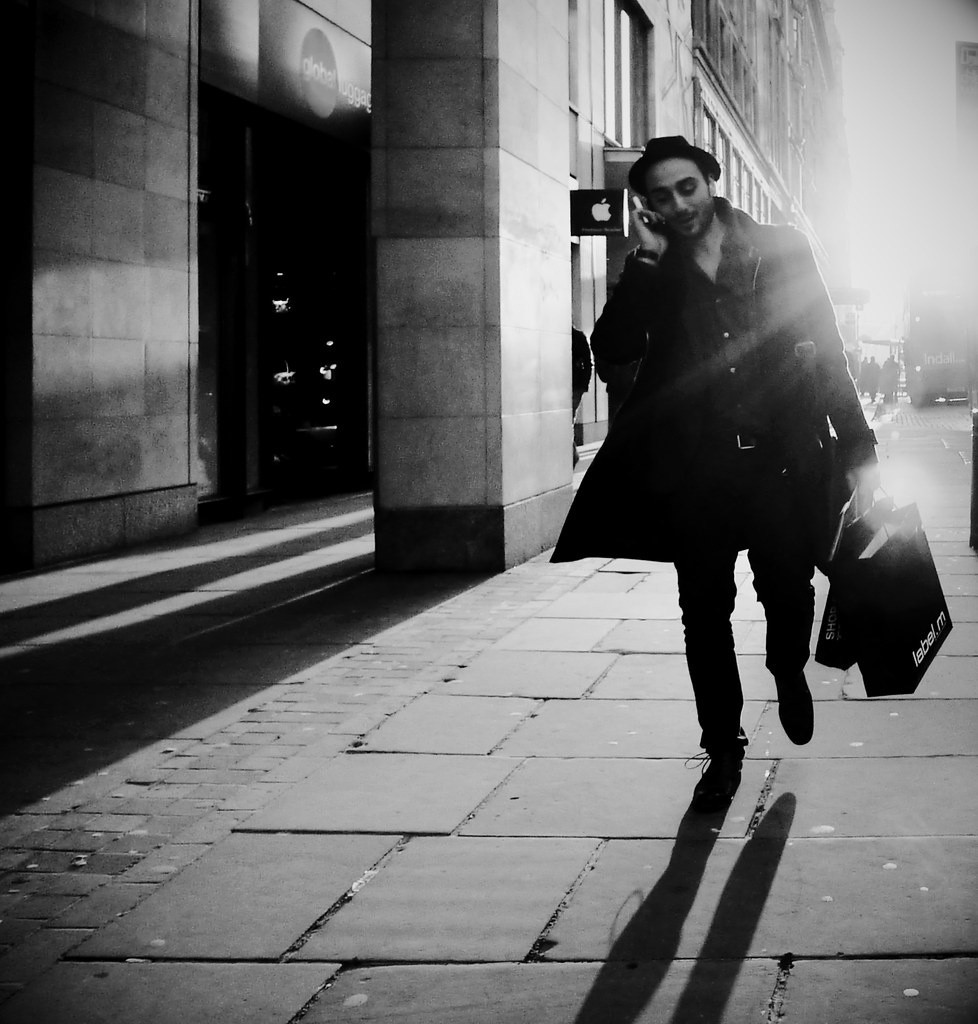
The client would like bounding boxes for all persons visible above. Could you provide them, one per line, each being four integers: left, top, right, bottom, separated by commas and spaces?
549, 136, 880, 812
572, 322, 592, 470
851, 354, 901, 403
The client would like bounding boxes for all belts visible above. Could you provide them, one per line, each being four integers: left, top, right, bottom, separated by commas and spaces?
683, 429, 790, 450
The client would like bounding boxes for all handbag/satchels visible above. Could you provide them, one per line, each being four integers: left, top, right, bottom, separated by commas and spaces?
814, 474, 954, 697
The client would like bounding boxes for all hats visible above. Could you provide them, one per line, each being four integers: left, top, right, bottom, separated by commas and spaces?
627, 136, 721, 196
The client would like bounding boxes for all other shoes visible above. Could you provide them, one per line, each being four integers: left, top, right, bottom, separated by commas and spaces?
691, 745, 746, 812
775, 671, 814, 745
